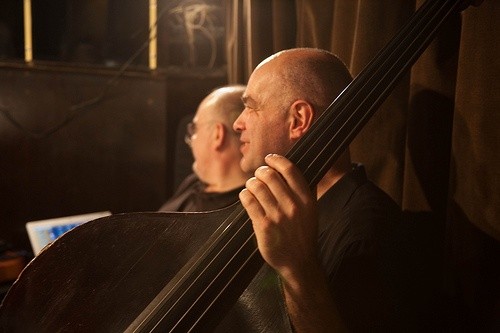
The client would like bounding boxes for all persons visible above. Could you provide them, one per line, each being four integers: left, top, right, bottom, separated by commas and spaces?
234, 47, 404, 333
153, 86, 254, 214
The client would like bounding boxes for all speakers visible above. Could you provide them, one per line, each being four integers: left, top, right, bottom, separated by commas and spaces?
0, 55, 225, 250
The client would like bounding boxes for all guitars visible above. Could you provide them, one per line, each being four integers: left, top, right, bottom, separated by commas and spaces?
0, 0, 469, 333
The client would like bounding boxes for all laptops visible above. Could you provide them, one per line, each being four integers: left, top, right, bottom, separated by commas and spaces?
25, 211, 111, 258
